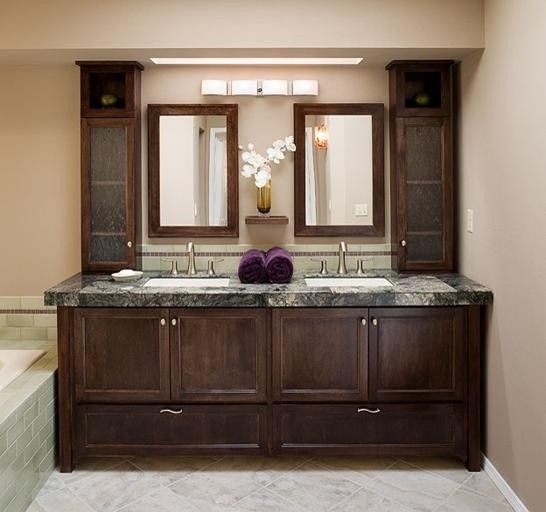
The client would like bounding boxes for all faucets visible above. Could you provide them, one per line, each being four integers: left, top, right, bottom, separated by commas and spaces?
186, 241, 196, 274
338, 241, 348, 274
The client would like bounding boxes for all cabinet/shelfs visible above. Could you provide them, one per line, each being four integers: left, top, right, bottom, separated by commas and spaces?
75, 60, 144, 273
385, 60, 455, 274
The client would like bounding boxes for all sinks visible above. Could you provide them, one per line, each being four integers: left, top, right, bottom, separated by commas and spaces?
141, 275, 230, 287
304, 275, 394, 287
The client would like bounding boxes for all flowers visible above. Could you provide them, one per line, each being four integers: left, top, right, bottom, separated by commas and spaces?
238, 135, 297, 188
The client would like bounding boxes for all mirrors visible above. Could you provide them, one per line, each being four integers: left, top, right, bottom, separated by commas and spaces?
294, 103, 385, 237
148, 104, 239, 238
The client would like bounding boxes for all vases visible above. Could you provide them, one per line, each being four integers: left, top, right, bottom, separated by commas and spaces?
257, 178, 271, 218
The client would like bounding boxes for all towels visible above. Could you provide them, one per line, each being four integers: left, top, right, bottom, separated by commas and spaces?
238, 249, 266, 284
265, 247, 293, 283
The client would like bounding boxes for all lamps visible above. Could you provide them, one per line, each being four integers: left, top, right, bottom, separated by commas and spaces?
200, 79, 320, 97
314, 126, 328, 150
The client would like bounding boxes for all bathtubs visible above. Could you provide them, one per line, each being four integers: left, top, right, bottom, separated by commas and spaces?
0, 348, 48, 389
